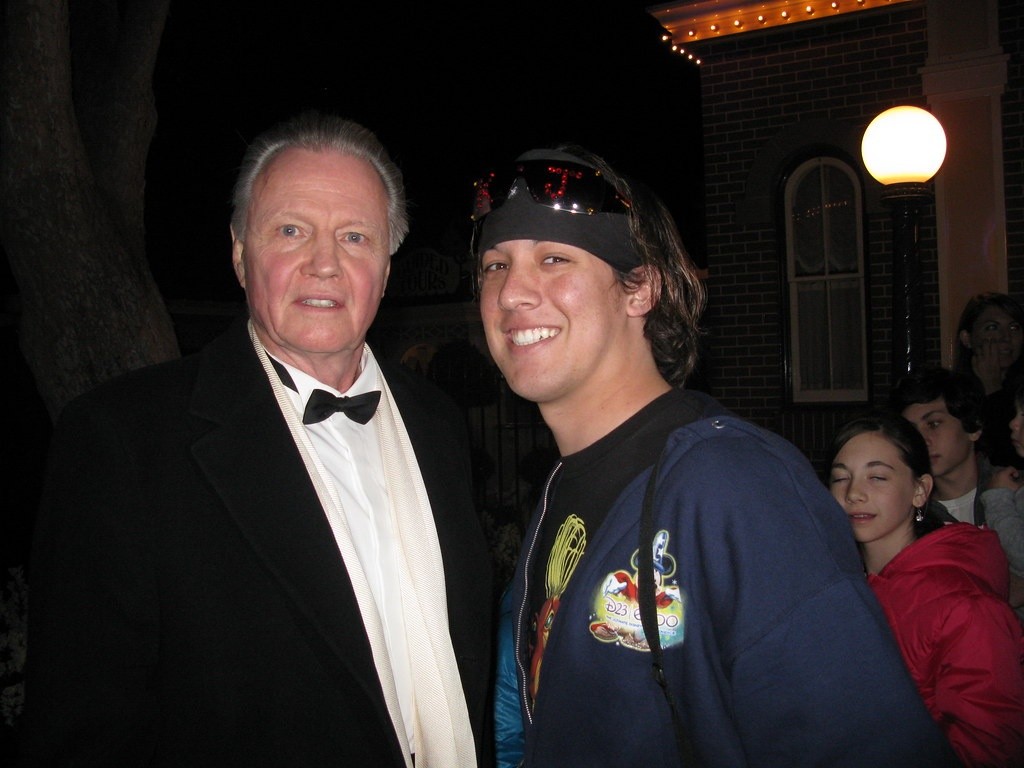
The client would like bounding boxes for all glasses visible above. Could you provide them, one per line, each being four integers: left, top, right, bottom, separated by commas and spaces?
466, 152, 636, 223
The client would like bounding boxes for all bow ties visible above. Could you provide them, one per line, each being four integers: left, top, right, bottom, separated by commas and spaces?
301, 380, 383, 429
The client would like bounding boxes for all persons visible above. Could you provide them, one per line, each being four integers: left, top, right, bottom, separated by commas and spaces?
11, 113, 491, 768
890, 369, 1024, 610
954, 291, 1024, 469
979, 382, 1024, 621
468, 147, 966, 768
828, 409, 1024, 768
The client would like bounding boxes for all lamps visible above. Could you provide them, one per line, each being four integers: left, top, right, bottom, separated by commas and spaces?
860, 107, 947, 201
